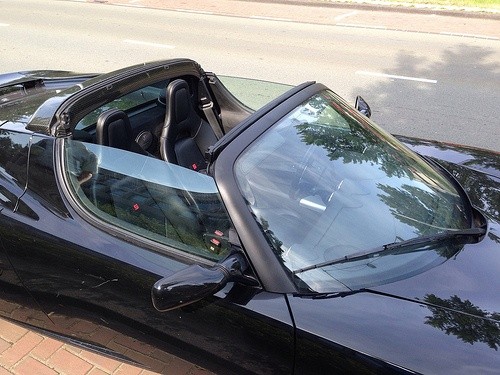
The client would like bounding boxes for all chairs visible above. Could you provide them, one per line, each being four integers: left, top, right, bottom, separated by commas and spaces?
158, 78, 233, 236
89, 110, 208, 254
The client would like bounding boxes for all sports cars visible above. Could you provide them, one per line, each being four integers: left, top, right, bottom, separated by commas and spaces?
0, 59, 500, 375
